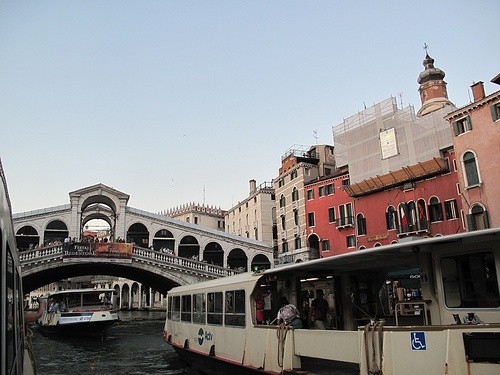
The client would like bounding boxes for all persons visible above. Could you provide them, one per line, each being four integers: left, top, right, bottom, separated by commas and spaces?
302, 290, 309, 329
307, 289, 336, 330
17, 234, 247, 273
277, 296, 302, 329
49, 300, 59, 313
207, 294, 213, 312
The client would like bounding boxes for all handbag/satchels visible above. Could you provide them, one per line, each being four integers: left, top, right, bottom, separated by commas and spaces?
311, 309, 318, 322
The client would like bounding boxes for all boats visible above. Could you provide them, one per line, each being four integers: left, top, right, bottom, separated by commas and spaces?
36, 287, 120, 326
162, 227, 500, 375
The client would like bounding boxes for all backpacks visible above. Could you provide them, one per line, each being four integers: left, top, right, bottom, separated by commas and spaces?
279, 304, 295, 323
